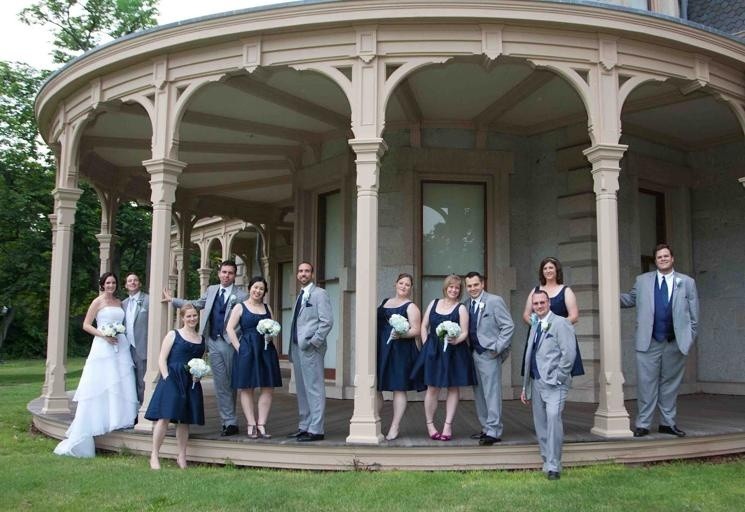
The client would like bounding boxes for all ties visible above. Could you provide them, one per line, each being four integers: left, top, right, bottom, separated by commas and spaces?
661, 275, 668, 303
536, 321, 541, 340
220, 289, 225, 306
293, 290, 304, 343
129, 297, 134, 312
471, 300, 476, 313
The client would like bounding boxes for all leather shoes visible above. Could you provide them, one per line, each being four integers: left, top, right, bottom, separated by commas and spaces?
288, 429, 307, 437
471, 432, 486, 439
549, 471, 560, 480
660, 425, 686, 435
221, 425, 238, 436
297, 433, 324, 441
479, 435, 501, 445
634, 428, 648, 437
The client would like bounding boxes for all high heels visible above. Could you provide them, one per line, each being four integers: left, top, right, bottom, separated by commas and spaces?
177, 457, 188, 469
256, 422, 272, 438
246, 423, 258, 439
150, 455, 161, 469
426, 421, 440, 441
385, 425, 399, 440
442, 420, 453, 440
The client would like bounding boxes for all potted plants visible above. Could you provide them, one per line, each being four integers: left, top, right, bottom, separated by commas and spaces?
228, 294, 237, 303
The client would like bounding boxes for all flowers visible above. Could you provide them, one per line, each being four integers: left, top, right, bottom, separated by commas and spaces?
479, 301, 486, 311
302, 291, 310, 300
101, 322, 126, 354
183, 357, 211, 389
255, 318, 283, 351
539, 320, 552, 333
435, 320, 462, 353
386, 312, 410, 346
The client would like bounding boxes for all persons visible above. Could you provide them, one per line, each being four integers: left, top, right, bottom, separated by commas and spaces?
460, 272, 515, 447
286, 261, 333, 442
138, 303, 205, 471
158, 260, 249, 435
617, 241, 701, 438
115, 274, 149, 401
406, 273, 479, 441
225, 275, 283, 440
519, 256, 585, 378
377, 273, 422, 441
517, 290, 577, 480
82, 272, 140, 430
